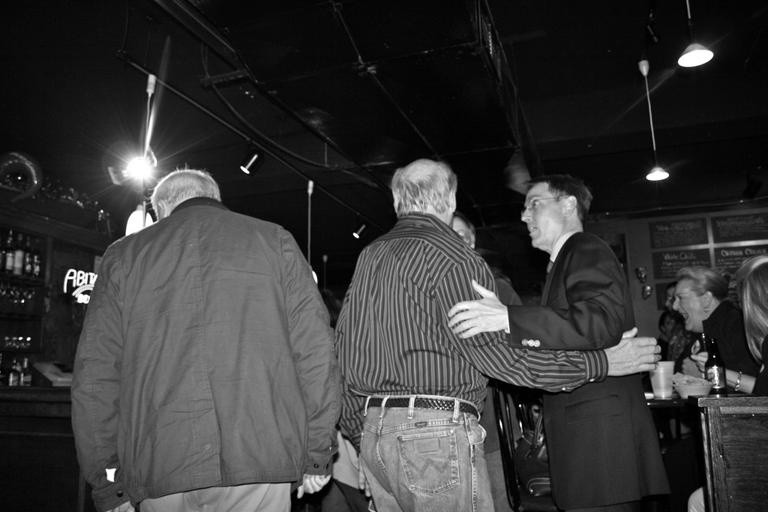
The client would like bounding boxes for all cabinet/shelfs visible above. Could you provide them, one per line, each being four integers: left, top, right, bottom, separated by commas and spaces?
1, 225, 46, 391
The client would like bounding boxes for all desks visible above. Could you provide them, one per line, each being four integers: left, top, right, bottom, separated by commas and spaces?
626, 395, 701, 509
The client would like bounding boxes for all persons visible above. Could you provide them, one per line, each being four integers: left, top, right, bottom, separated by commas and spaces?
445, 171, 675, 512
71, 165, 344, 510
448, 210, 524, 306
654, 255, 768, 397
332, 153, 666, 510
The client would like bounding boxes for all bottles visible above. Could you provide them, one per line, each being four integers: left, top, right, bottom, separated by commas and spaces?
0, 230, 44, 279
705, 336, 727, 397
698, 333, 709, 379
0, 336, 34, 387
0, 285, 37, 305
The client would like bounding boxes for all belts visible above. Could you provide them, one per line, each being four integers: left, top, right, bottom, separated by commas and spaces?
367, 398, 478, 418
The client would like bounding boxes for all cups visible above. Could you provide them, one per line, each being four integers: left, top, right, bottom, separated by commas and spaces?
649, 360, 675, 398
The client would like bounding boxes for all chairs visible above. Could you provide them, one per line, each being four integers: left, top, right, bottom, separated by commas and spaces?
695, 387, 768, 512
489, 384, 558, 511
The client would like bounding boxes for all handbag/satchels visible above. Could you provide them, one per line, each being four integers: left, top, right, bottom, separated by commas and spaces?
513, 430, 552, 497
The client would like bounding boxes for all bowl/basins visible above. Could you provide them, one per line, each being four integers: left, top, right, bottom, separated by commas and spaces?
675, 384, 713, 398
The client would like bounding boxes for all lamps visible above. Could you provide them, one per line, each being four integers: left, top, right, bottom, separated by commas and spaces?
302, 176, 318, 289
110, 62, 371, 241
637, 59, 669, 182
674, 1, 714, 68
